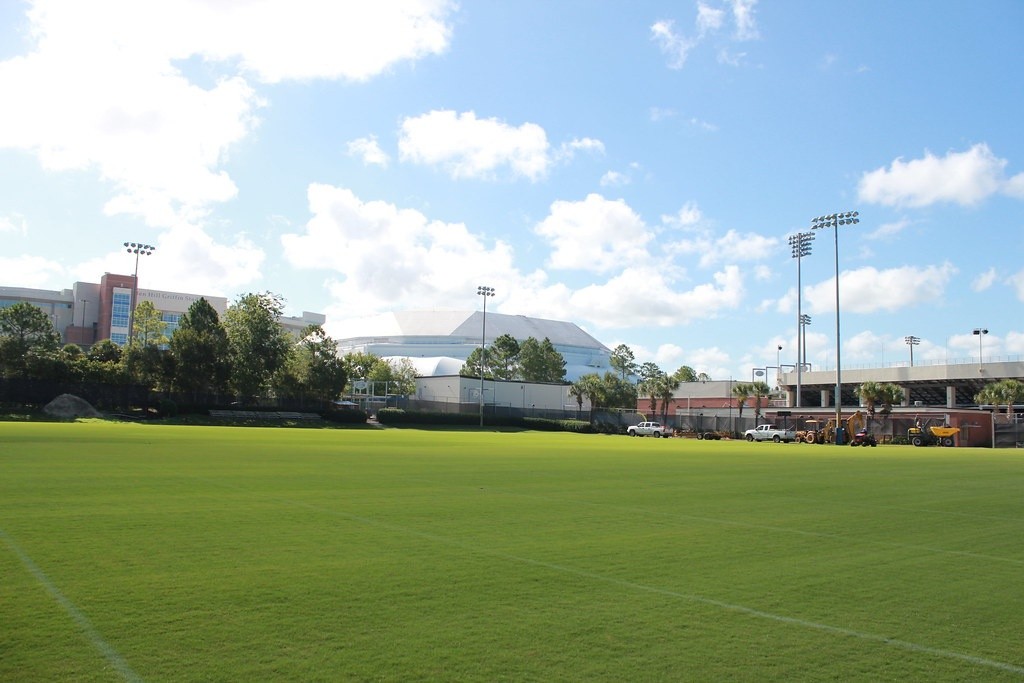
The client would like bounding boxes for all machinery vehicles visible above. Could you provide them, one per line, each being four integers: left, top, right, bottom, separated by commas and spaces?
850, 431, 877, 447
795, 409, 864, 444
908, 416, 960, 446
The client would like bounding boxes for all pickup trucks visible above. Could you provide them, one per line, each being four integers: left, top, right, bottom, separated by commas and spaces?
745, 424, 796, 444
627, 422, 673, 439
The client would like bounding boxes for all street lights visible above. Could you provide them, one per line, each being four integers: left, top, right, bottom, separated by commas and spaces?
476, 285, 495, 429
799, 314, 811, 365
119, 241, 157, 413
973, 328, 988, 363
811, 211, 860, 410
905, 335, 920, 368
788, 230, 816, 405
